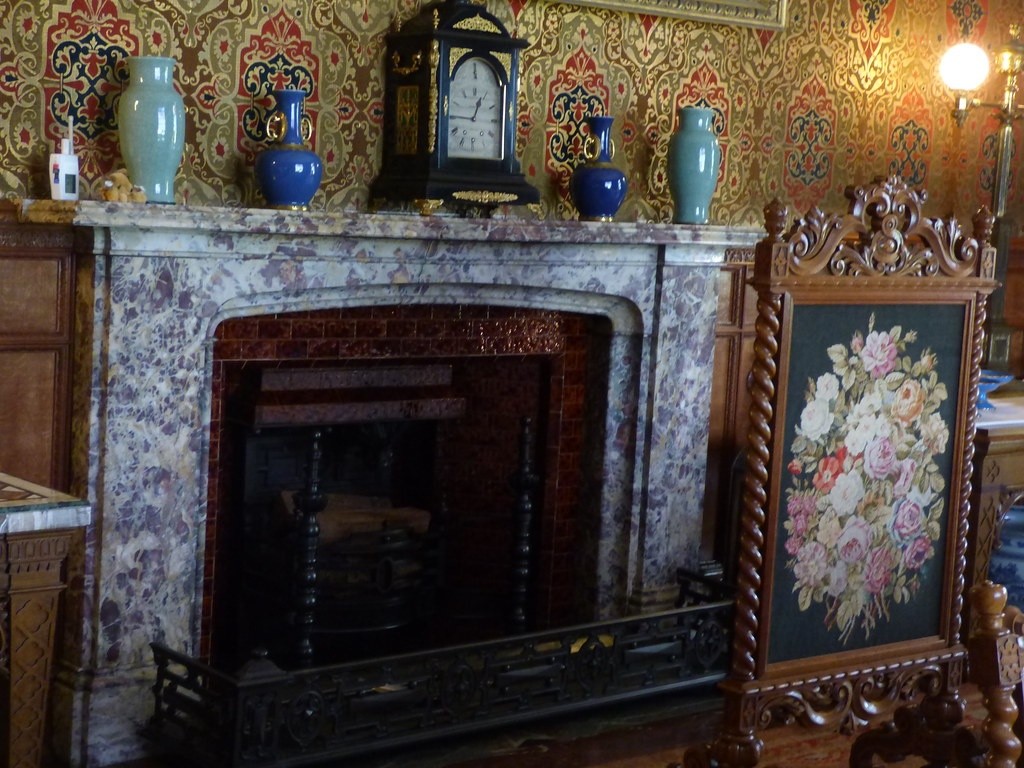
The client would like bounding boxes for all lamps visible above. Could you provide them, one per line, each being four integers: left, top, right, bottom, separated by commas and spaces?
939, 21, 1024, 373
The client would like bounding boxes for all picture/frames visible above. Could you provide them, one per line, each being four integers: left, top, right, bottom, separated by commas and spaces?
687, 174, 1003, 768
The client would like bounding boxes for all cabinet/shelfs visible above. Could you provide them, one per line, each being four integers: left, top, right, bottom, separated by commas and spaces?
0, 471, 93, 768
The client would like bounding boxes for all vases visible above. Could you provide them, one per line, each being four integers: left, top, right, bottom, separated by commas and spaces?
117, 56, 187, 203
253, 89, 324, 212
666, 107, 721, 222
569, 116, 629, 223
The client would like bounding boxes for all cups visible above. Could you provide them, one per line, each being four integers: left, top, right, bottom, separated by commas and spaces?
48, 154, 79, 200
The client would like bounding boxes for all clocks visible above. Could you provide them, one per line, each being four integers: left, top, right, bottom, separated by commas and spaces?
364, 0, 541, 218
